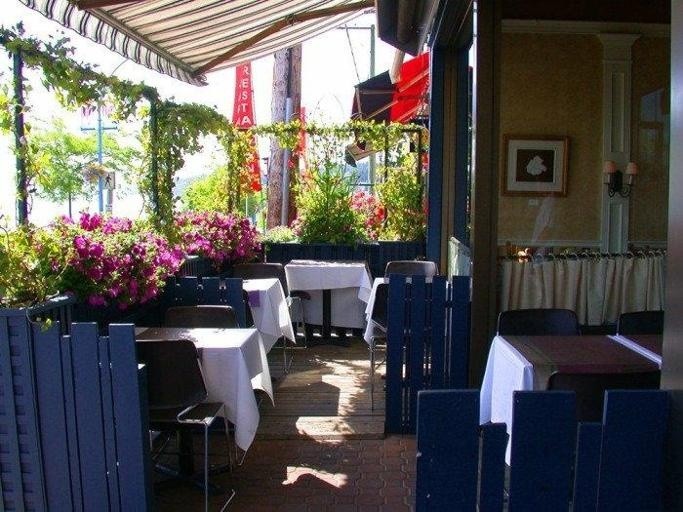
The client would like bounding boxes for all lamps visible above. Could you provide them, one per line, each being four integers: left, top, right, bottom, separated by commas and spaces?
603, 160, 639, 198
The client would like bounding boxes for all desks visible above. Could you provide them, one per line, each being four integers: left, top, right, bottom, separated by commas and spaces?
176, 278, 297, 355
134, 327, 275, 465
284, 259, 374, 348
479, 332, 662, 467
364, 276, 452, 385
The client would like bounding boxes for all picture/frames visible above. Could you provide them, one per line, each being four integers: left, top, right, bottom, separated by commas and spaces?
502, 134, 570, 197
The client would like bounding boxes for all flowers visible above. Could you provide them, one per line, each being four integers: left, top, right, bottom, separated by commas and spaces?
291, 216, 308, 235
31, 208, 261, 309
352, 189, 386, 242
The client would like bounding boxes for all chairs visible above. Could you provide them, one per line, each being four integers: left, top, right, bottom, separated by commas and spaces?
234, 262, 295, 373
372, 284, 446, 414
219, 288, 254, 327
166, 305, 263, 409
496, 306, 665, 421
371, 260, 438, 370
135, 339, 236, 512
